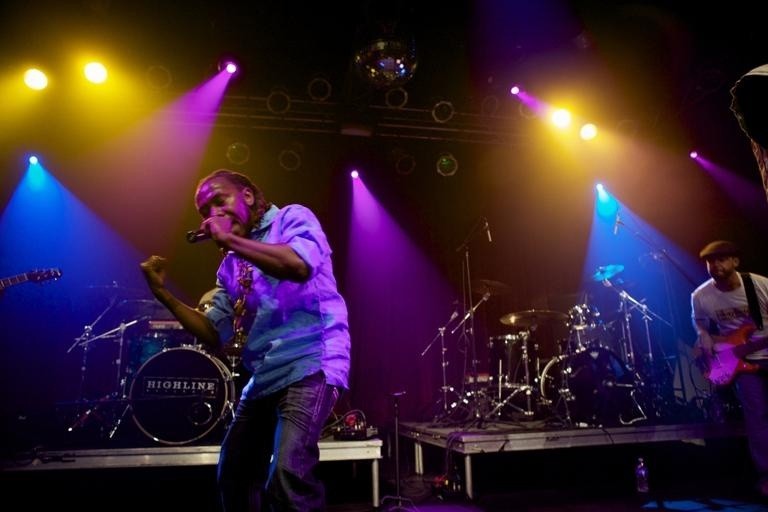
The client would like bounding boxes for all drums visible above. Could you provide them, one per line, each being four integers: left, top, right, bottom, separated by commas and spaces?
129, 344, 236, 450
490, 336, 541, 423
550, 343, 642, 429
134, 321, 200, 368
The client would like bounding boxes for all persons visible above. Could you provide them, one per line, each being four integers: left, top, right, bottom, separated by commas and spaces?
140, 166, 352, 511
689, 241, 767, 500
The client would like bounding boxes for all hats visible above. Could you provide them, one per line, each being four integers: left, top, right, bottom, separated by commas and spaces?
700, 241, 736, 257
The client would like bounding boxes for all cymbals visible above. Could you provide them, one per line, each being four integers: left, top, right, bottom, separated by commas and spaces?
472, 275, 515, 303
120, 299, 165, 309
87, 285, 147, 298
504, 307, 569, 329
585, 264, 628, 285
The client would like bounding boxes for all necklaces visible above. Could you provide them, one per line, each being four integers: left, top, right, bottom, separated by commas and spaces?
219, 231, 255, 377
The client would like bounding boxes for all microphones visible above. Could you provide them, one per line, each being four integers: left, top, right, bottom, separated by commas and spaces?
186, 228, 211, 244
137, 314, 152, 322
387, 391, 407, 397
480, 214, 493, 242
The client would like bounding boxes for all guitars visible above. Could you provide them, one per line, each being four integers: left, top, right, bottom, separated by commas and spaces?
0, 266, 62, 294
694, 322, 768, 385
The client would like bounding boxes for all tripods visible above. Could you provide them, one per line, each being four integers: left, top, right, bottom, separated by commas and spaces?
56, 295, 136, 448
421, 299, 573, 430
